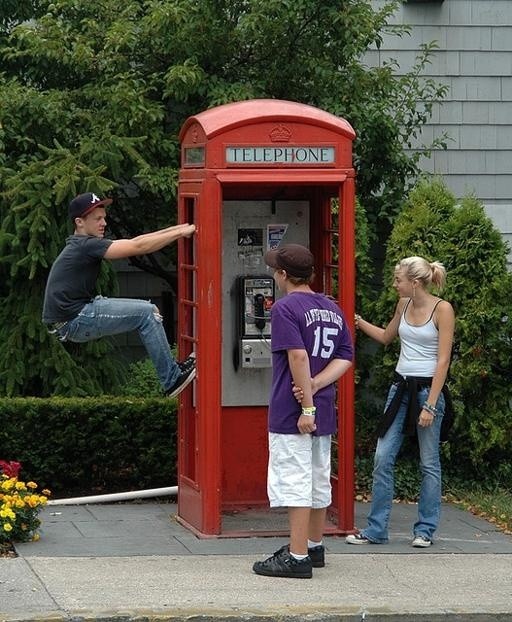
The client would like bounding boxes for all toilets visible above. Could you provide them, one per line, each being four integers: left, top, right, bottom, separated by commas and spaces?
237, 274, 275, 367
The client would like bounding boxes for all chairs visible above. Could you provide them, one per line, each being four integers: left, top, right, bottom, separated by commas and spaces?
251, 544, 326, 578
412, 536, 432, 548
346, 533, 371, 545
165, 353, 197, 399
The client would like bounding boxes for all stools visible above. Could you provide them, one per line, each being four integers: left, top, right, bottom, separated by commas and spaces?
50, 321, 68, 331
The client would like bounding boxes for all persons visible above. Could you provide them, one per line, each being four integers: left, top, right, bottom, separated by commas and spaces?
344, 256, 455, 548
252, 244, 354, 579
41, 191, 197, 400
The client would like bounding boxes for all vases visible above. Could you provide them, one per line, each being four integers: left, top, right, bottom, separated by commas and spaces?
1, 457, 54, 554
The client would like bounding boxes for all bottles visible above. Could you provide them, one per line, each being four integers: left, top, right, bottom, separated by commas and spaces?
69, 191, 113, 222
264, 244, 315, 278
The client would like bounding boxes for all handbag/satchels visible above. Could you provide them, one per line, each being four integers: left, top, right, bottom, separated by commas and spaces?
354, 314, 362, 330
422, 400, 438, 417
300, 407, 317, 416
